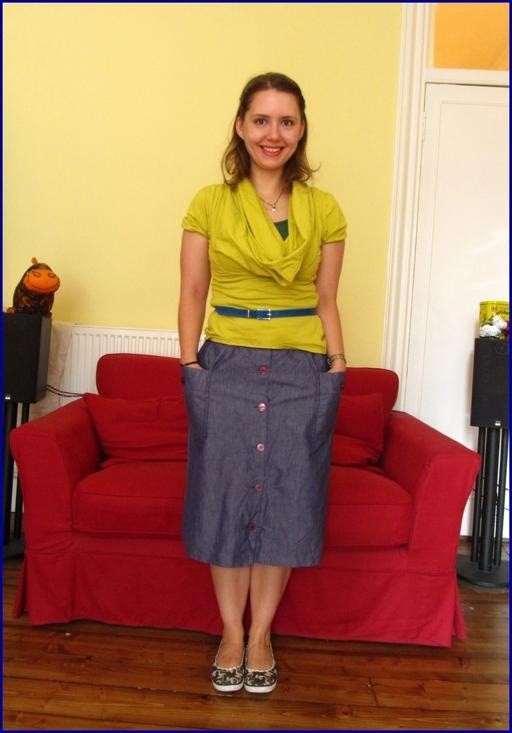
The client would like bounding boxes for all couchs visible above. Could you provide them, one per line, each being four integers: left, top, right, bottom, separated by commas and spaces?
9, 350, 487, 650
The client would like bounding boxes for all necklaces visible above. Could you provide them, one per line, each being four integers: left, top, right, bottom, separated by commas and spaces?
254, 178, 291, 211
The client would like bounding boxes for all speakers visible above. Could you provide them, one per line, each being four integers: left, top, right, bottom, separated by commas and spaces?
455, 337, 511, 587
0, 311, 53, 562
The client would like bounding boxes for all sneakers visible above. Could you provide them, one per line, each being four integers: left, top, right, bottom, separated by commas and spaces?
244, 639, 278, 694
211, 640, 245, 693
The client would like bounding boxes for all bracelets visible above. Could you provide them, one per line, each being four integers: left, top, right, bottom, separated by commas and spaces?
326, 352, 348, 367
180, 359, 199, 367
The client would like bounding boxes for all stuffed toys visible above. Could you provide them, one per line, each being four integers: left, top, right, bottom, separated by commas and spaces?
7, 255, 62, 319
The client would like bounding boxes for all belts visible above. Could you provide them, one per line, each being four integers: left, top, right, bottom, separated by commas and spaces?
218, 306, 316, 318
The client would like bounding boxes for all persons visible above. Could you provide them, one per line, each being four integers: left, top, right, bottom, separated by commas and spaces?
175, 67, 353, 696
